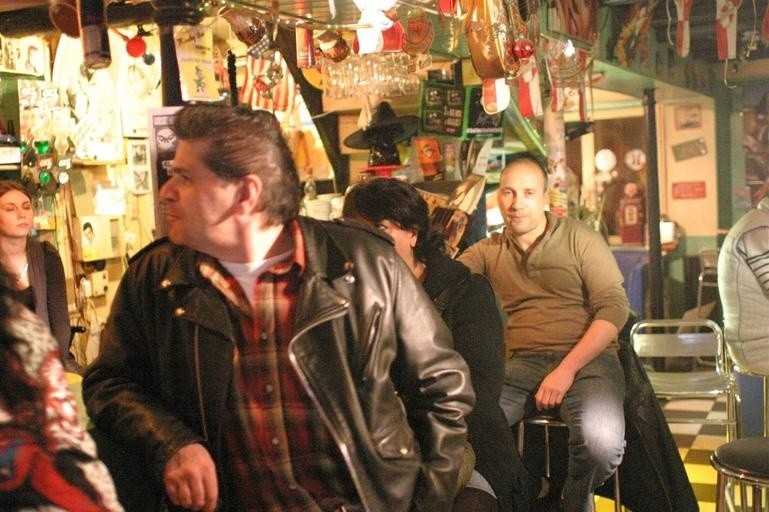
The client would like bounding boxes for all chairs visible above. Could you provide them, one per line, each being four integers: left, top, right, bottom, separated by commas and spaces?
629, 317, 738, 512
693, 246, 721, 367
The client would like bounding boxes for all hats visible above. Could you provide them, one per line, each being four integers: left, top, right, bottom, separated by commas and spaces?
344, 101, 420, 151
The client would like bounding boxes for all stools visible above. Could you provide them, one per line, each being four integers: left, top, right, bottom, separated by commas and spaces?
709, 436, 769, 512
518, 411, 621, 512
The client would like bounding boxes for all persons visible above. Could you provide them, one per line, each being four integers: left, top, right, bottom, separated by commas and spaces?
81, 100, 476, 512
1, 260, 126, 512
0, 179, 71, 369
452, 151, 631, 512
716, 178, 769, 438
341, 176, 531, 511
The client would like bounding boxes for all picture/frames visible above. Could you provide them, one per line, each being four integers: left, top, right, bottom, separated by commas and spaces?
416, 78, 506, 143
146, 105, 186, 244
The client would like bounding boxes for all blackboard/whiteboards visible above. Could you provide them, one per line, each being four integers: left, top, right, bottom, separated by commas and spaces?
417, 77, 509, 141
